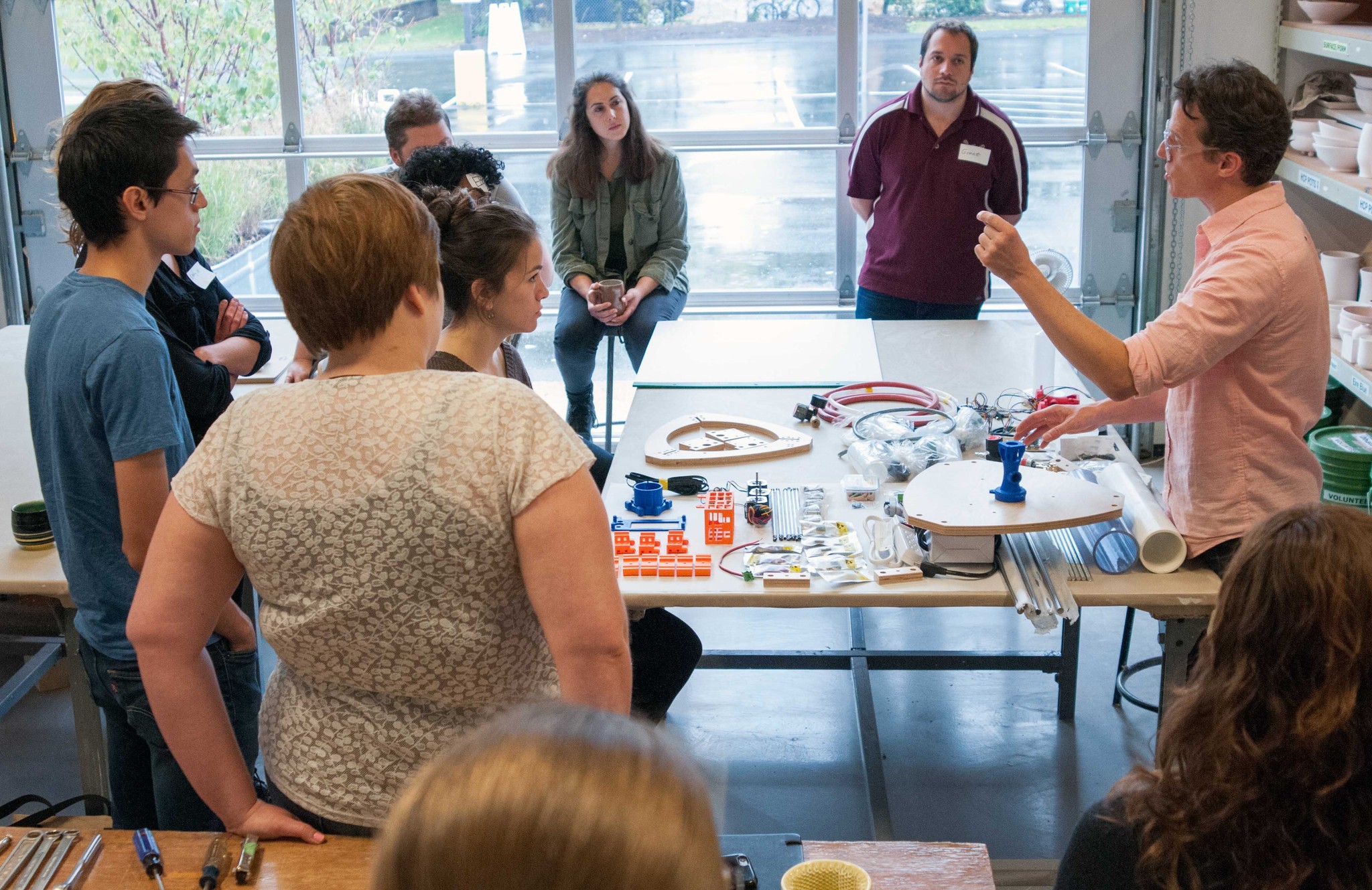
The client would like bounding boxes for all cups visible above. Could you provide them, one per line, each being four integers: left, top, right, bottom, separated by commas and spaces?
1319, 250, 1372, 371
10, 499, 57, 551
782, 858, 873, 890
586, 279, 626, 317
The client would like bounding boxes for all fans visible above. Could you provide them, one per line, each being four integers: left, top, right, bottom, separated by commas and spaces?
1030, 250, 1074, 297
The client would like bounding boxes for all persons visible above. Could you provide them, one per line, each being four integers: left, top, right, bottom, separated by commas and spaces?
25, 78, 273, 830
546, 71, 691, 442
282, 88, 701, 727
846, 17, 1028, 321
371, 702, 728, 890
973, 58, 1331, 689
1053, 500, 1372, 890
127, 171, 633, 843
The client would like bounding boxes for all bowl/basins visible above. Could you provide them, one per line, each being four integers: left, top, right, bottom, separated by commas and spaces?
1288, 117, 1372, 179
1298, 0, 1360, 24
1350, 69, 1372, 119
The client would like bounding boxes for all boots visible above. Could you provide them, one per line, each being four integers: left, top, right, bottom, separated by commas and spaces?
563, 383, 599, 443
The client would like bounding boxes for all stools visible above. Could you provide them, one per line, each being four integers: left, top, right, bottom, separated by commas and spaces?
589, 325, 625, 453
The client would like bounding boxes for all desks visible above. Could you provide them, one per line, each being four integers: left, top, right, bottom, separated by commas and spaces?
597, 320, 1225, 838
0, 310, 307, 812
0, 827, 998, 890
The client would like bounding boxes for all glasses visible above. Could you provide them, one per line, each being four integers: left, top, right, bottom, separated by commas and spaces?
1164, 119, 1234, 162
138, 182, 200, 204
721, 853, 758, 890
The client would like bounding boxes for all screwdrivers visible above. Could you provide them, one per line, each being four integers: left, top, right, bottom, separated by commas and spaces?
197, 833, 229, 890
132, 827, 166, 890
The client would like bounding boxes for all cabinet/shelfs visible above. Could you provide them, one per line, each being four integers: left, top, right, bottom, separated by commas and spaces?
1267, 0, 1372, 407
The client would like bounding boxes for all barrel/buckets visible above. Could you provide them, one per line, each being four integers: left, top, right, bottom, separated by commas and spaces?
1302, 374, 1372, 512
